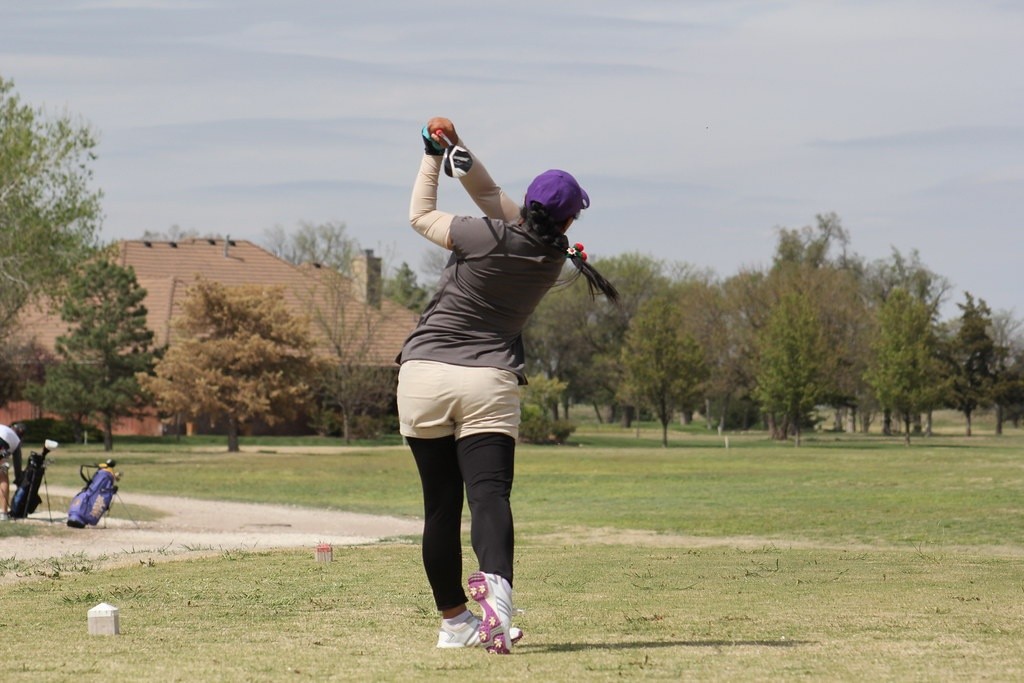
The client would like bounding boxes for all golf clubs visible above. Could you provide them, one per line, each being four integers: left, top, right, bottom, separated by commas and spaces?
431, 125, 473, 179
0, 439, 123, 523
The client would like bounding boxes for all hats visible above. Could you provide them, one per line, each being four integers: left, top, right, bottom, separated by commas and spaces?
526, 169, 591, 223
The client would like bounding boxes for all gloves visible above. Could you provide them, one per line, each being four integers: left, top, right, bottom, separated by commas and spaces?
421, 124, 445, 156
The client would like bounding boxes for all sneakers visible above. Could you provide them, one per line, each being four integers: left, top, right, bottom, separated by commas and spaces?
436, 612, 523, 648
467, 572, 511, 654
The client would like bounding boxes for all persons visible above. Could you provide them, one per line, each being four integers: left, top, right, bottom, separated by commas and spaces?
0, 421, 26, 521
394, 117, 621, 655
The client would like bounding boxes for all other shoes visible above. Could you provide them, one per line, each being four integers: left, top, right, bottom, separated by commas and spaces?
0, 512, 8, 521
